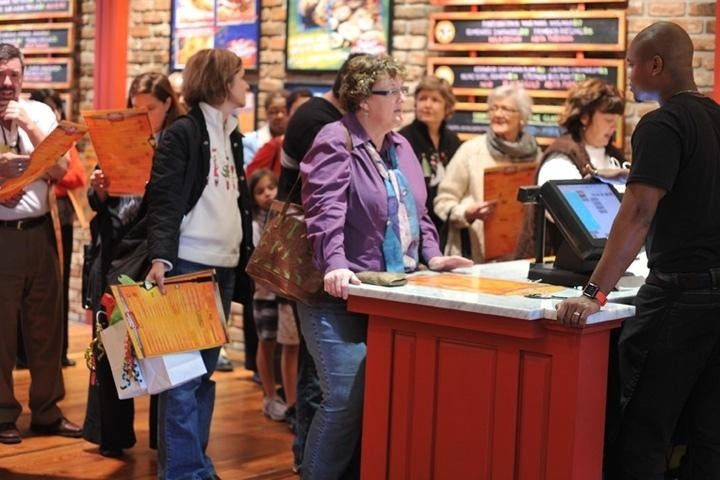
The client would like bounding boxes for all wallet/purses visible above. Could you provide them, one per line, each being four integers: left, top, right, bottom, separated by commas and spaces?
356, 271, 407, 287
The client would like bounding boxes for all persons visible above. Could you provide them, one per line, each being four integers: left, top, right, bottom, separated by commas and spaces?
141, 48, 252, 480
396, 75, 464, 231
82, 72, 190, 457
556, 21, 719, 480
15, 87, 88, 371
295, 53, 476, 480
512, 77, 628, 259
433, 84, 544, 265
0, 43, 83, 443
215, 53, 367, 473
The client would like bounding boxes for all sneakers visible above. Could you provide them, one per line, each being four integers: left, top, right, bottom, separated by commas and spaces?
262, 394, 289, 421
215, 346, 233, 371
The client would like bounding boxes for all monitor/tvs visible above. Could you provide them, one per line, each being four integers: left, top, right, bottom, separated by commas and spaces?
540, 178, 621, 262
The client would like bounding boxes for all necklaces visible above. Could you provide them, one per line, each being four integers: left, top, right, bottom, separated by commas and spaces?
672, 89, 704, 98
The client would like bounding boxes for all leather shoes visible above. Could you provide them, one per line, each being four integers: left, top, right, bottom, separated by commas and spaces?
0, 424, 22, 444
99, 443, 122, 457
31, 417, 84, 438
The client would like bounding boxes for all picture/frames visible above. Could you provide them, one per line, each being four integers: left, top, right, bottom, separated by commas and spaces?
283, 0, 395, 76
167, 0, 262, 75
281, 81, 335, 99
177, 83, 260, 136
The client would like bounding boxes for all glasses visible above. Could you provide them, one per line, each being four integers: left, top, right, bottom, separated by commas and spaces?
486, 106, 522, 113
370, 86, 409, 96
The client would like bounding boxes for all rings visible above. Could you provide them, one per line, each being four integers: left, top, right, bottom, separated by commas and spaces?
591, 170, 597, 176
573, 311, 580, 317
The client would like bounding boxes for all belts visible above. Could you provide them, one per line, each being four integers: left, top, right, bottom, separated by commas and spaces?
0, 213, 49, 230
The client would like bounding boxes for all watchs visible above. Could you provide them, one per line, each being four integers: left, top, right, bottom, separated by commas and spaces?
582, 283, 608, 306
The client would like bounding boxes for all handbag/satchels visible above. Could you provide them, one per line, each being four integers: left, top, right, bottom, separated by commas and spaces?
101, 215, 153, 309
100, 318, 148, 401
138, 350, 209, 395
83, 241, 102, 308
245, 199, 326, 308
